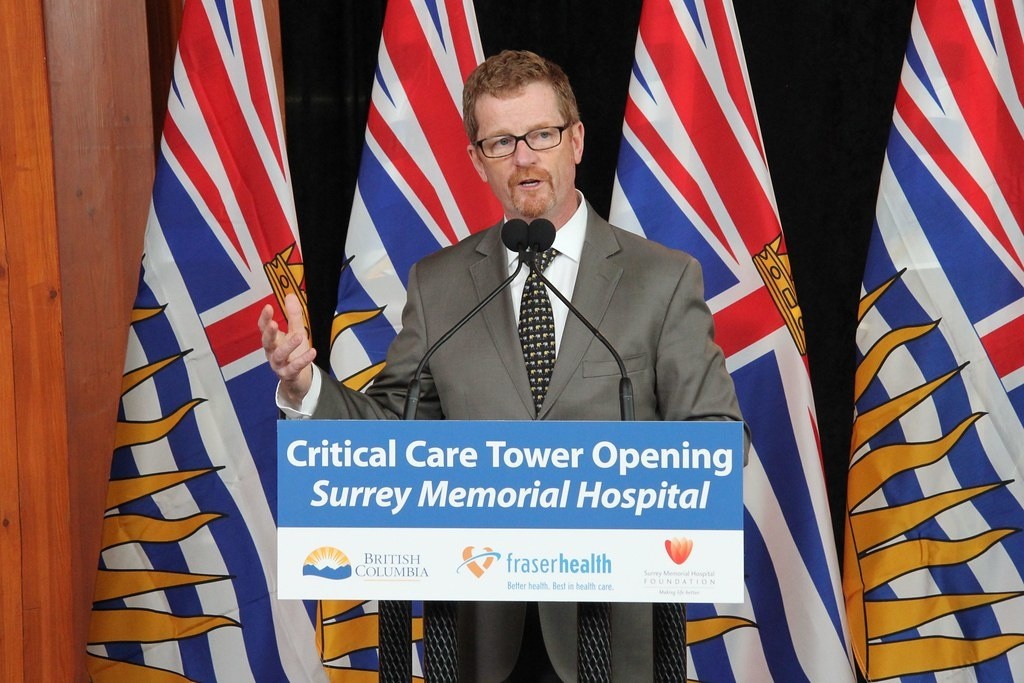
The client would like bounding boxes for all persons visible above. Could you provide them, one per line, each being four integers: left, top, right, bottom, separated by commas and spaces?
259, 51, 749, 683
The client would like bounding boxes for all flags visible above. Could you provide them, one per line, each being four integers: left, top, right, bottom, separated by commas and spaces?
84, 1, 1024, 683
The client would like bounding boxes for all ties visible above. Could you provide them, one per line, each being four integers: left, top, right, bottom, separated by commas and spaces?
520, 248, 560, 420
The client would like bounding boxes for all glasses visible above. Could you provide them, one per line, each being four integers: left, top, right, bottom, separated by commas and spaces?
471, 123, 570, 158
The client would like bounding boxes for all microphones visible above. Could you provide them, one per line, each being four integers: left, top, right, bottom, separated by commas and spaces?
529, 218, 636, 421
400, 218, 528, 421
